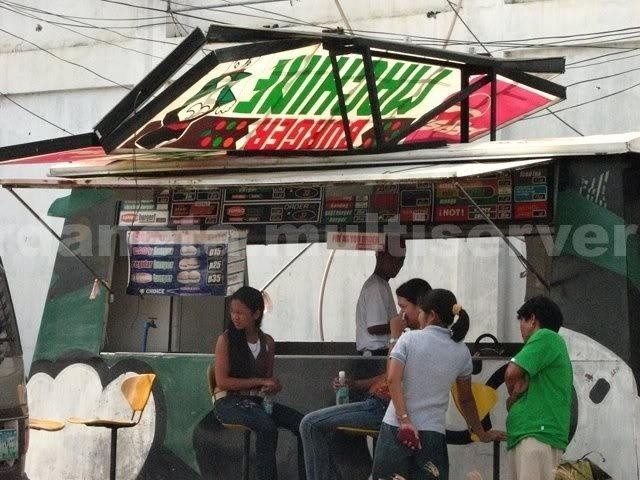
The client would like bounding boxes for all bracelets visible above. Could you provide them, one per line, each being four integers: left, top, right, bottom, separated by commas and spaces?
396, 414, 409, 419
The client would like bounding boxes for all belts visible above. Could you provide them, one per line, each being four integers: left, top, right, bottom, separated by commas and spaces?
211, 388, 262, 405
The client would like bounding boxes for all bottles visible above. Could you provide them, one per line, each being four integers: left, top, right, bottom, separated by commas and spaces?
263, 391, 272, 414
335, 371, 349, 405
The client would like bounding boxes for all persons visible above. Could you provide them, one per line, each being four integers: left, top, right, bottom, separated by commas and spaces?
504, 295, 573, 480
370, 288, 510, 480
299, 277, 433, 479
212, 286, 305, 479
356, 236, 406, 356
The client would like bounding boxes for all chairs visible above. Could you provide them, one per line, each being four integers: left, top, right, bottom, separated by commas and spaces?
452, 382, 512, 478
27, 418, 65, 431
208, 362, 257, 479
67, 373, 156, 480
326, 427, 378, 480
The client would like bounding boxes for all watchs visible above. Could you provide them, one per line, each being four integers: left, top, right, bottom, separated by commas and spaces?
385, 337, 397, 345
